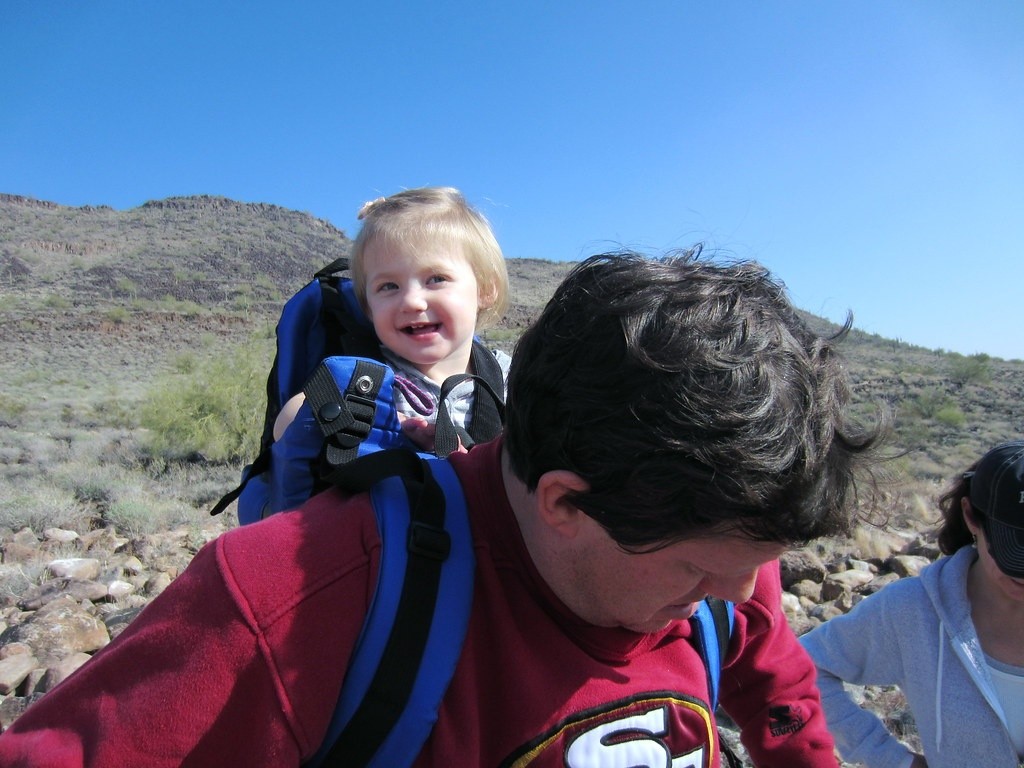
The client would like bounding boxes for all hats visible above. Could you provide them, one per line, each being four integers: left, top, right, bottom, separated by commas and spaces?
969, 440, 1024, 578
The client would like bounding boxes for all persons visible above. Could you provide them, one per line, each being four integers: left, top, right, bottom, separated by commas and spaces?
272, 183, 518, 510
790, 441, 1024, 768
0, 244, 915, 768
